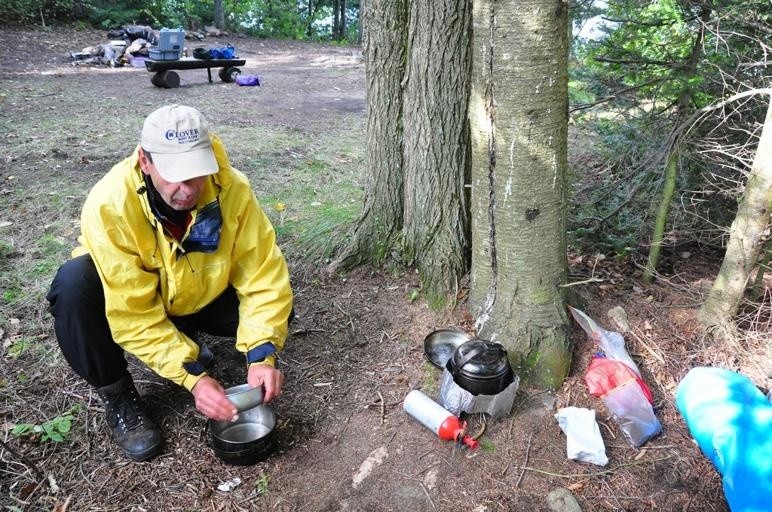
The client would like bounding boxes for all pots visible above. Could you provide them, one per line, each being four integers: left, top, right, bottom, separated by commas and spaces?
209, 405, 277, 466
445, 338, 514, 397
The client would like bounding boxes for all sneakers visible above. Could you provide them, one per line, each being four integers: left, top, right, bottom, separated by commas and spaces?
175, 321, 215, 374
95, 371, 164, 462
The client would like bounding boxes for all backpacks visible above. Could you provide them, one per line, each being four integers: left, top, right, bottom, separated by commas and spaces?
193, 47, 214, 60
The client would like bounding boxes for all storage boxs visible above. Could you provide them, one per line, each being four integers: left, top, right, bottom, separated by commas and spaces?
148, 48, 181, 61
157, 26, 185, 50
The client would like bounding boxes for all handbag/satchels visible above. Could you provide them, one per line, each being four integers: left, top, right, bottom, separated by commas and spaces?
210, 42, 236, 59
236, 74, 260, 86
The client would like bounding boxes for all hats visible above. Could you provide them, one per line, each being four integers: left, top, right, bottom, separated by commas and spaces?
140, 103, 219, 184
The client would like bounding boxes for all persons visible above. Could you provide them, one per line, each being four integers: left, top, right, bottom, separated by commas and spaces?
45, 104, 294, 463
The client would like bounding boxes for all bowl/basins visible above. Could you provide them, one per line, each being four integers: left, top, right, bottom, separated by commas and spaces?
221, 382, 265, 414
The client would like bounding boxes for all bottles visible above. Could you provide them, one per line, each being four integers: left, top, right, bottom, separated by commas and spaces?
402, 389, 479, 450
